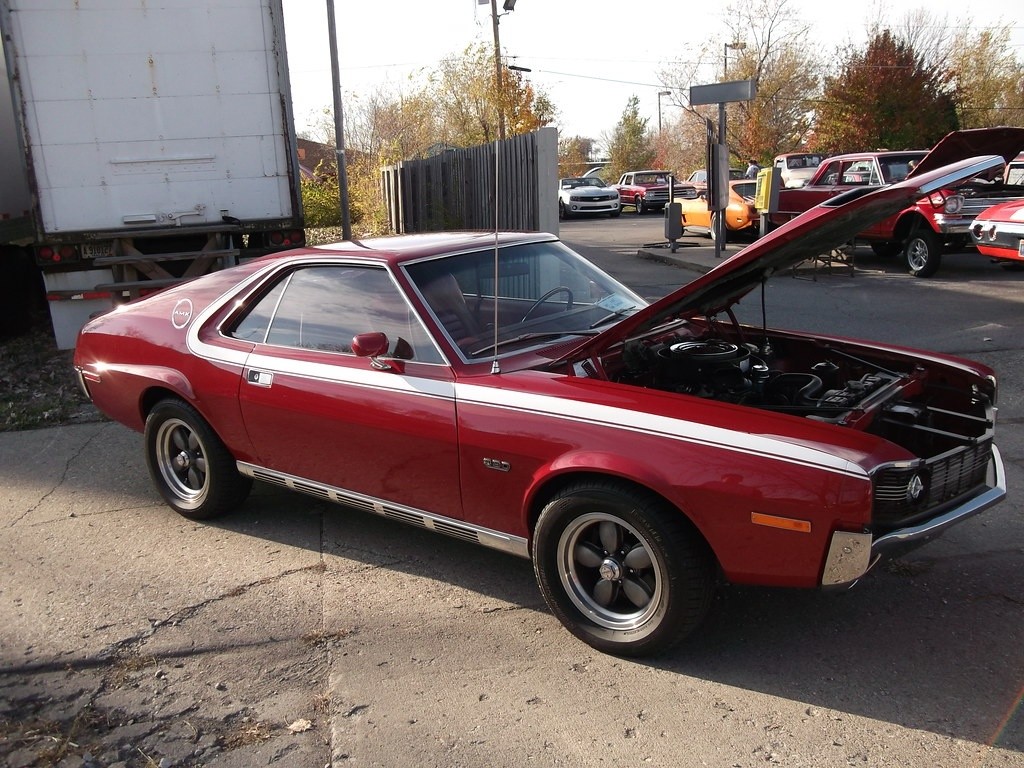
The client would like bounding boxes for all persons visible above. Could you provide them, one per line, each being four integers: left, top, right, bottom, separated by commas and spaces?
746, 160, 764, 180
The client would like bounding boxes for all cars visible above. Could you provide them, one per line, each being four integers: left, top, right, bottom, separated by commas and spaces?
558, 175, 622, 216
613, 153, 835, 243
74, 153, 1009, 660
774, 125, 1024, 275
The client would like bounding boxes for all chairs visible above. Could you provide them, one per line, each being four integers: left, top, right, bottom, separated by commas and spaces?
419, 273, 482, 344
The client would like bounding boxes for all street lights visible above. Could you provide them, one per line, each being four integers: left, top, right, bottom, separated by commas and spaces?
657, 90, 672, 134
724, 39, 748, 83
476, 0, 517, 139
